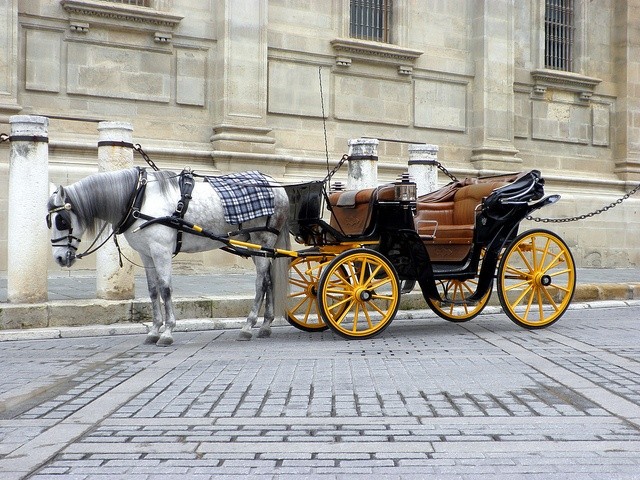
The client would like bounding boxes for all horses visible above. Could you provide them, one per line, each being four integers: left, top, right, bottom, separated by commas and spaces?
46, 167, 291, 347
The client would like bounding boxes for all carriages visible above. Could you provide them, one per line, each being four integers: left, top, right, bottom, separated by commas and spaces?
46, 166, 576, 347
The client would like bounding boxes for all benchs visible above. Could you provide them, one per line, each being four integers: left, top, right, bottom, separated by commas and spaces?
413, 182, 512, 238
